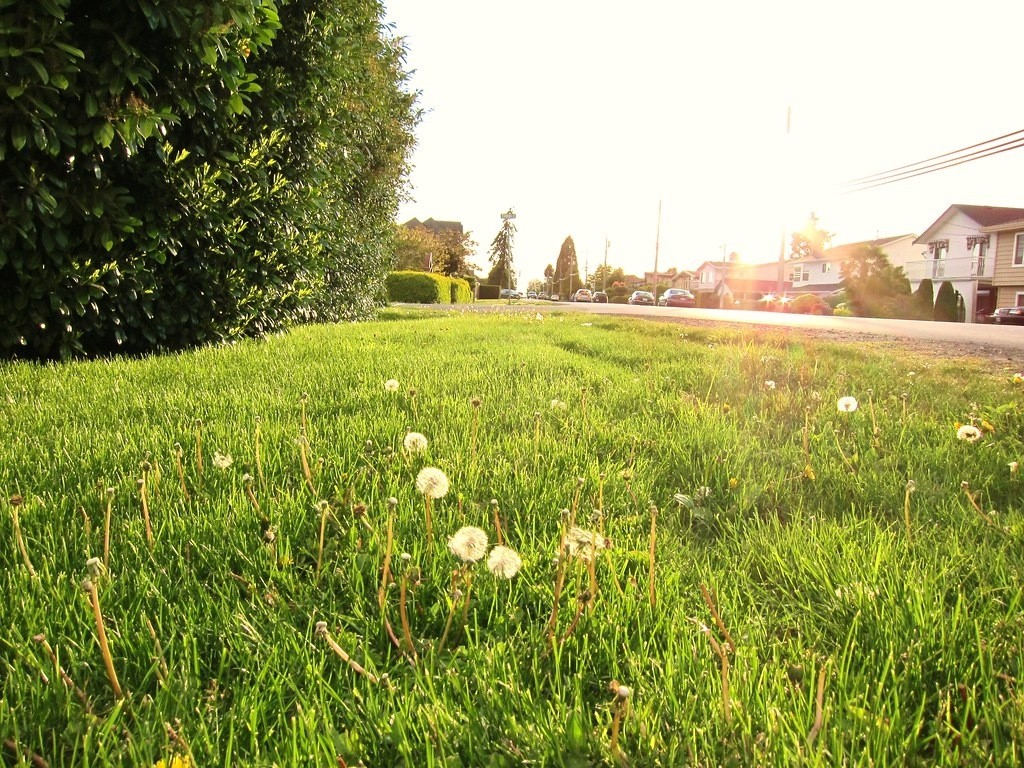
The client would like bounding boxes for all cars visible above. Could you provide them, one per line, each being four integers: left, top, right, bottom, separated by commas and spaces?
592, 292, 608, 303
501, 289, 520, 300
551, 295, 559, 301
658, 288, 695, 307
538, 292, 547, 300
574, 289, 591, 303
628, 291, 654, 306
985, 307, 1024, 326
527, 292, 537, 299
